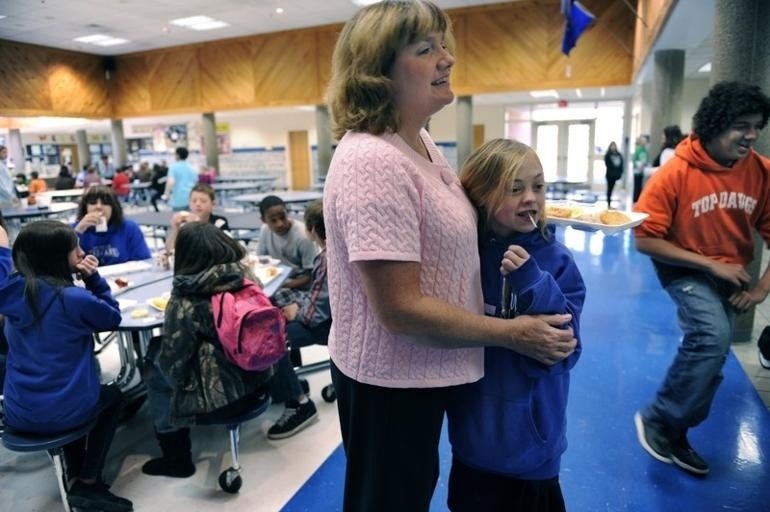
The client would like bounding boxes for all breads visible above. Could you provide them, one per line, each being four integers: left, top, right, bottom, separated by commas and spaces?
154, 293, 171, 308
115, 277, 127, 286
267, 266, 277, 276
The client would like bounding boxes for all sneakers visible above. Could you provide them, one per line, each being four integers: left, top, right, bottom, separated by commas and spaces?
267, 381, 318, 439
634, 411, 710, 474
68, 480, 132, 511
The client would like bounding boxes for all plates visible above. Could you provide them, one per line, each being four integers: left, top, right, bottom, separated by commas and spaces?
258, 258, 281, 267
146, 297, 166, 313
540, 202, 650, 237
98, 260, 149, 277
116, 298, 137, 312
253, 267, 284, 287
109, 281, 134, 295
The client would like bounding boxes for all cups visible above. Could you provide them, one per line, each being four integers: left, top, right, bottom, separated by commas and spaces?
151, 252, 160, 267
94, 216, 108, 233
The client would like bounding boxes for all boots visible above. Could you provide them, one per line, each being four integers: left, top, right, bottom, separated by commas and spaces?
142, 427, 196, 478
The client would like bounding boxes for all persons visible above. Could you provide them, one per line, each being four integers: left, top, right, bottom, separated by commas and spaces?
141, 221, 279, 477
1, 145, 46, 247
0, 224, 13, 283
632, 80, 770, 475
256, 195, 319, 307
1, 220, 133, 512
165, 185, 228, 251
602, 122, 685, 205
268, 203, 330, 438
756, 324, 770, 370
55, 155, 146, 202
448, 135, 587, 512
152, 147, 212, 211
73, 182, 151, 269
318, 0, 578, 511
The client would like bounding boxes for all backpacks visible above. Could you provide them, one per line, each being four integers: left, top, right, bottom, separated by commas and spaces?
212, 276, 287, 372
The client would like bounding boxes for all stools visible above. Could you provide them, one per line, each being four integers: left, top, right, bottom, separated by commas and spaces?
194, 388, 273, 494
0, 419, 97, 512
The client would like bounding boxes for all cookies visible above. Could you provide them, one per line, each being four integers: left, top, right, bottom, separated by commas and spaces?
545, 206, 630, 224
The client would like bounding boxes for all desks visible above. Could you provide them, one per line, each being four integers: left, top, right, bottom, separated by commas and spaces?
0, 172, 323, 424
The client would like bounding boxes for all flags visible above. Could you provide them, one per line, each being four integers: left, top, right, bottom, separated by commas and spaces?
560, 0, 597, 58
